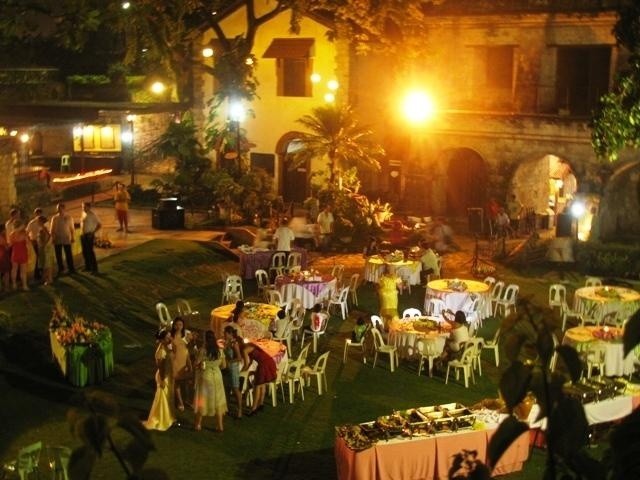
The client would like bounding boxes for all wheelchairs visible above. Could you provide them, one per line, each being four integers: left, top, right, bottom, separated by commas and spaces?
492, 223, 512, 242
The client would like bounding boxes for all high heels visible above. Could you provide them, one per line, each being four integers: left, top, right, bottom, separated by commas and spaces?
246, 409, 259, 418
255, 404, 264, 412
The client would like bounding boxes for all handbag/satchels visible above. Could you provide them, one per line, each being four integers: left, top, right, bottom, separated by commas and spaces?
199, 360, 206, 370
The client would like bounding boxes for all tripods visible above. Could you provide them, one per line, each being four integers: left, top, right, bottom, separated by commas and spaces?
495, 231, 512, 261
459, 247, 494, 275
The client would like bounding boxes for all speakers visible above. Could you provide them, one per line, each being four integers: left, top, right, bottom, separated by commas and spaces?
468, 208, 484, 234
151, 206, 185, 229
556, 213, 571, 236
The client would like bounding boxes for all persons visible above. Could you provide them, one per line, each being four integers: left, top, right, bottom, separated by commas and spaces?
302, 304, 327, 339
430, 308, 468, 371
362, 238, 439, 319
488, 194, 524, 240
112, 180, 132, 233
38, 170, 51, 188
589, 206, 601, 240
0, 201, 102, 293
351, 316, 366, 344
253, 190, 334, 267
139, 301, 290, 432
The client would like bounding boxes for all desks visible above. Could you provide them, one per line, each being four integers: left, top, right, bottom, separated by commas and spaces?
51, 324, 113, 388
335, 406, 530, 480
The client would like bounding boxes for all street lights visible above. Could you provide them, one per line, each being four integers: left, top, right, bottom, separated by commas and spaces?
229, 102, 247, 175
